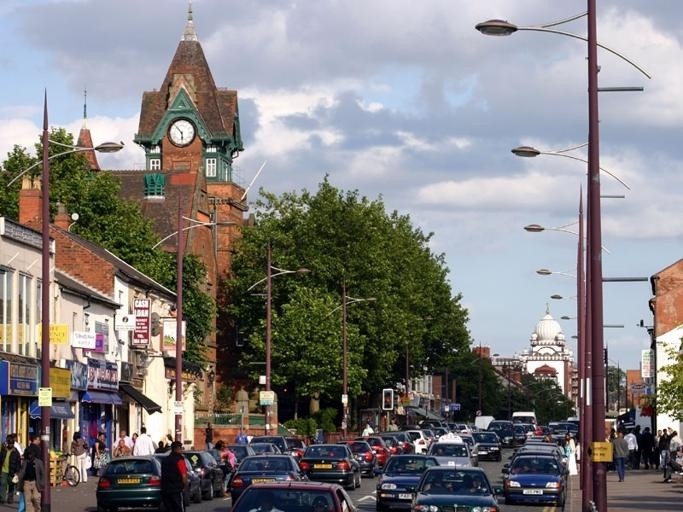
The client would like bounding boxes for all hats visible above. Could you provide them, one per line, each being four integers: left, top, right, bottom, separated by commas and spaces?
7, 439, 14, 444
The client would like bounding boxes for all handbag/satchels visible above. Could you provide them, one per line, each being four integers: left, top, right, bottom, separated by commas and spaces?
219, 461, 232, 474
12, 475, 19, 484
18, 493, 25, 512
83, 444, 87, 448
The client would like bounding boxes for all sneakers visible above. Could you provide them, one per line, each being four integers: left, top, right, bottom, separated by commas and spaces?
220, 492, 229, 497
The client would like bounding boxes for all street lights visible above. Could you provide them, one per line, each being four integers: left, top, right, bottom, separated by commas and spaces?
320, 278, 378, 441
152, 188, 237, 443
475, 1, 654, 510
4, 88, 124, 512
509, 143, 652, 500
247, 241, 312, 433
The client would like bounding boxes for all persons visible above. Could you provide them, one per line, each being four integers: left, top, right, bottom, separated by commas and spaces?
204, 420, 214, 449
362, 421, 374, 436
208, 439, 238, 497
469, 475, 487, 492
311, 496, 327, 511
386, 418, 399, 432
156, 440, 192, 511
430, 475, 453, 490
246, 491, 284, 511
235, 424, 249, 444
15, 445, 45, 511
0, 427, 173, 503
542, 422, 682, 484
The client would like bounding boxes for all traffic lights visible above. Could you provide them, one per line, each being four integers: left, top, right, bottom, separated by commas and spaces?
381, 389, 394, 411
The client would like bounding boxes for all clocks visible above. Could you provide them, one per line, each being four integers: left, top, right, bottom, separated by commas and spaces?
167, 118, 198, 148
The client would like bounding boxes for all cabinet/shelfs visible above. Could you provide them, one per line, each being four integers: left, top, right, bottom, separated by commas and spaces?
49, 456, 64, 489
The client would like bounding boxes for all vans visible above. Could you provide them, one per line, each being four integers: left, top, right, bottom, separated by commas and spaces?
510, 410, 536, 428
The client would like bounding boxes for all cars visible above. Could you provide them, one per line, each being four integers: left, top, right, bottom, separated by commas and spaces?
230, 481, 362, 512
302, 443, 363, 491
99, 457, 165, 511
409, 464, 501, 512
329, 419, 579, 478
374, 455, 443, 512
154, 435, 308, 506
500, 453, 570, 511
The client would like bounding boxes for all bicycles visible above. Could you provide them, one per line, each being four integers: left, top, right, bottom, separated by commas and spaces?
58, 450, 81, 490
